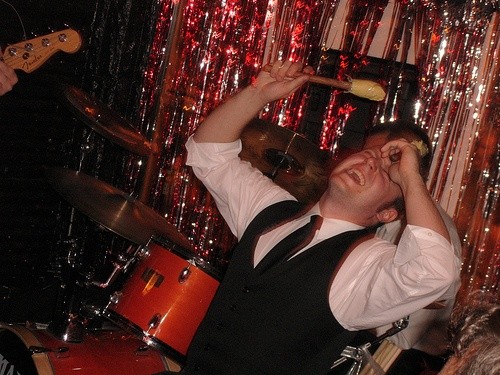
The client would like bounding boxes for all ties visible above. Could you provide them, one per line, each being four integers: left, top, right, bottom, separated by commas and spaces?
254, 214, 323, 274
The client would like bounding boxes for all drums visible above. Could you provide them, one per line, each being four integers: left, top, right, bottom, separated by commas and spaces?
102, 235, 222, 357
1, 314, 173, 375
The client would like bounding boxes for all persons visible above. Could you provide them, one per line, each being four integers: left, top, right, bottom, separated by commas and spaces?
327, 119, 461, 375
160, 59, 461, 375
0, 52, 19, 96
435, 290, 499, 375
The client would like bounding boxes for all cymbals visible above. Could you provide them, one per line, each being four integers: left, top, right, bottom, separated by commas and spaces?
42, 165, 195, 261
60, 80, 146, 150
237, 117, 332, 206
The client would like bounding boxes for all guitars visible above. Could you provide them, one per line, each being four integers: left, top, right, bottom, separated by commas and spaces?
0, 29, 83, 74
